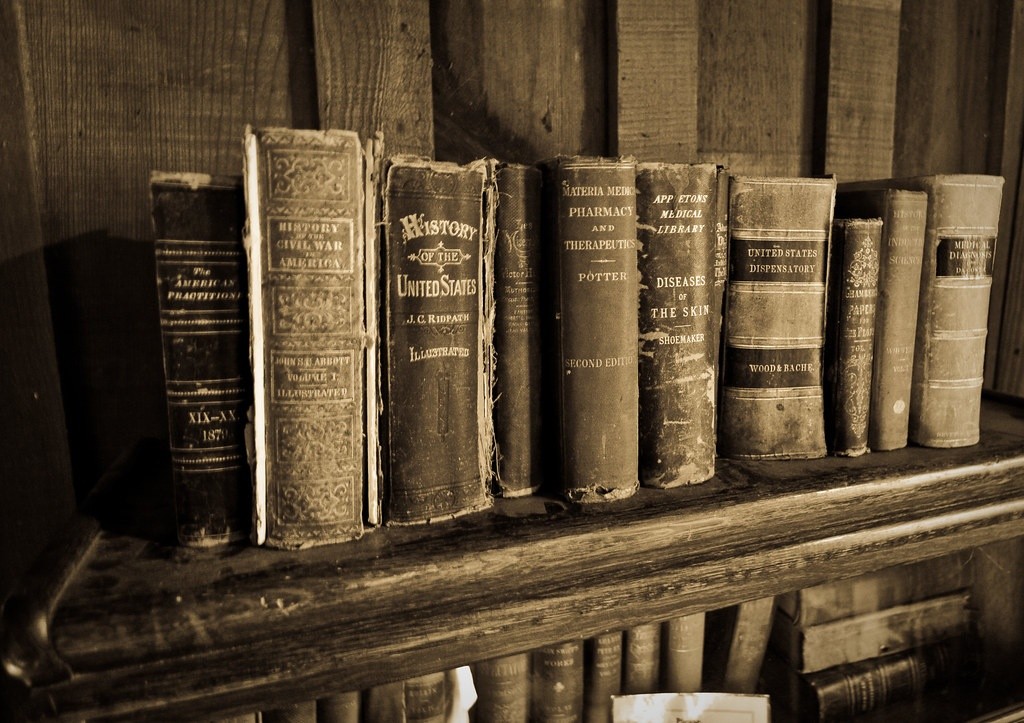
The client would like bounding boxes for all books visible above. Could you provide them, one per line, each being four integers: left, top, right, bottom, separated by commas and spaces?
151, 127, 1007, 555
205, 547, 976, 723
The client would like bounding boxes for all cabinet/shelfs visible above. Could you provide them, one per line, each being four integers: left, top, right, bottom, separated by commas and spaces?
0, 0, 1024, 723
